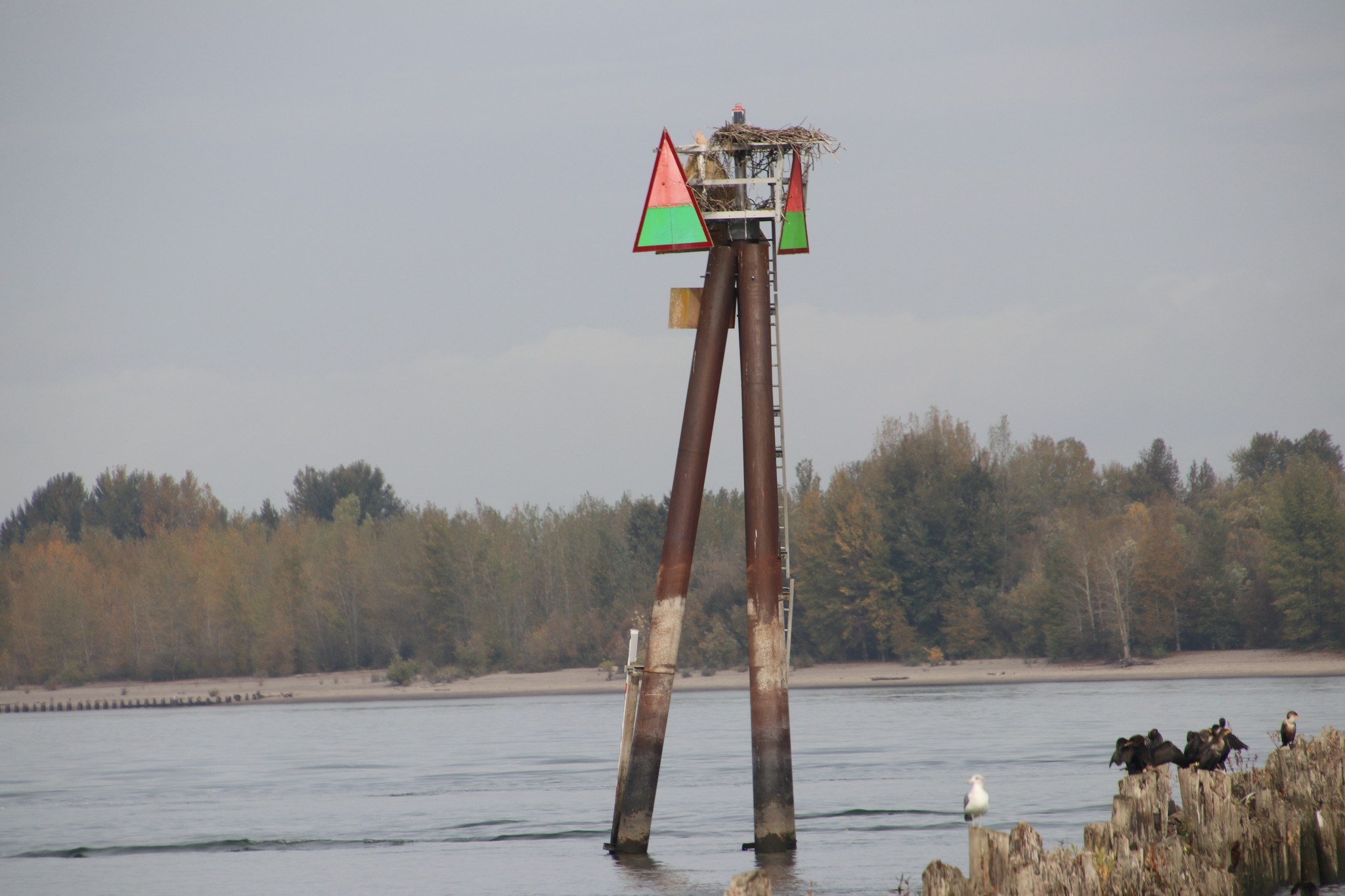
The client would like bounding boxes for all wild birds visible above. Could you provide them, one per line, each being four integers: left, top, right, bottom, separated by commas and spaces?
1278, 709, 1300, 745
1104, 715, 1247, 771
962, 773, 989, 828
